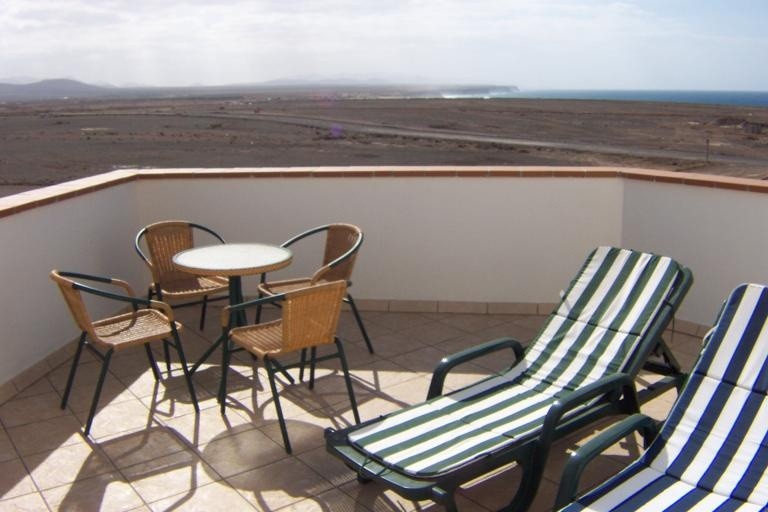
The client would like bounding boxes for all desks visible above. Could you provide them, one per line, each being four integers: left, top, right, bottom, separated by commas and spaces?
173, 242, 294, 403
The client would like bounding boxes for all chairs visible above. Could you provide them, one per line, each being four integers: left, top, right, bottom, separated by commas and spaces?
135, 220, 226, 374
254, 222, 375, 379
324, 246, 694, 512
49, 268, 200, 435
221, 279, 361, 454
554, 282, 768, 512
